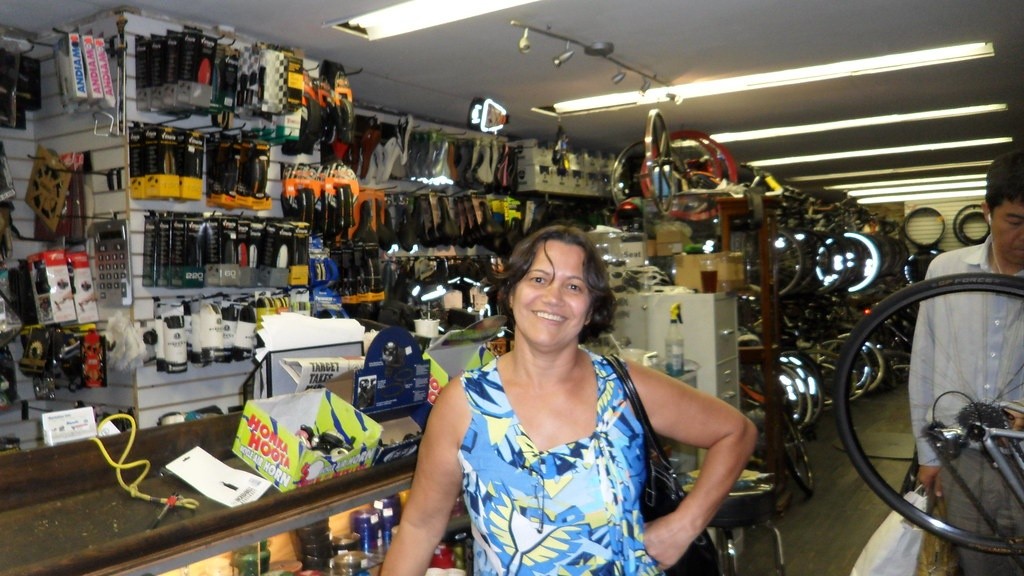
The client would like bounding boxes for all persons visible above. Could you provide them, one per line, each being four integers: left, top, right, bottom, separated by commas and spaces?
377, 224, 759, 576
907, 146, 1024, 576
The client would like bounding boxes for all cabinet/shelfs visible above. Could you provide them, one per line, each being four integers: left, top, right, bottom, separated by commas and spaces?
0, 409, 471, 576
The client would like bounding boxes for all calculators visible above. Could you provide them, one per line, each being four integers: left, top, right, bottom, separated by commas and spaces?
89, 219, 134, 307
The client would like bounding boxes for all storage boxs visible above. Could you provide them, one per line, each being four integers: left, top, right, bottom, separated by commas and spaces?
128, 23, 309, 289
26, 30, 118, 326
673, 251, 746, 292
230, 313, 509, 496
587, 231, 646, 268
655, 223, 693, 256
508, 138, 616, 198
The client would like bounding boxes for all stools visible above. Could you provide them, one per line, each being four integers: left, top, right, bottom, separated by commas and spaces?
682, 477, 787, 576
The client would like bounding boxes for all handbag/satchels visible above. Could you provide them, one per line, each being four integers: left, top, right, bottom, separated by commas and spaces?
603, 354, 724, 576
917, 500, 960, 576
850, 483, 928, 576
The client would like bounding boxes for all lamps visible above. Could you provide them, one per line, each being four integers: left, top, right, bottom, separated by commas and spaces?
640, 75, 651, 95
553, 41, 575, 67
518, 27, 532, 54
612, 65, 626, 85
666, 93, 684, 106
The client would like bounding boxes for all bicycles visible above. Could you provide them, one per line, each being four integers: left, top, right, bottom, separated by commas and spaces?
832, 271, 1024, 572
609, 109, 919, 433
